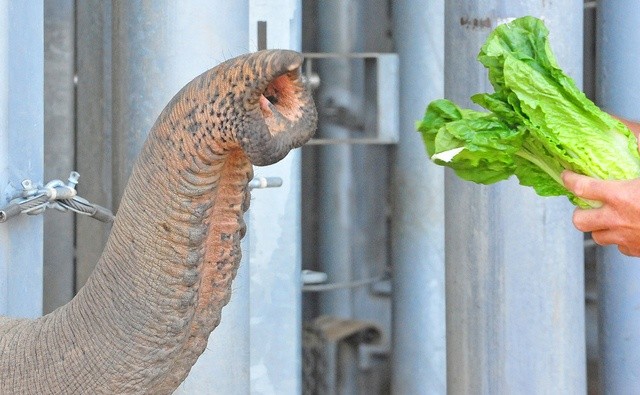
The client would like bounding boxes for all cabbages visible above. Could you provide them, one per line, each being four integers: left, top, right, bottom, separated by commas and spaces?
415, 15, 640, 209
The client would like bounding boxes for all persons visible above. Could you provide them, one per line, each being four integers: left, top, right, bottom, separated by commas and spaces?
561, 113, 640, 258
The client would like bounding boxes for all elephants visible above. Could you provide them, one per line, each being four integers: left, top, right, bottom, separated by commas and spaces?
0, 48, 319, 395
